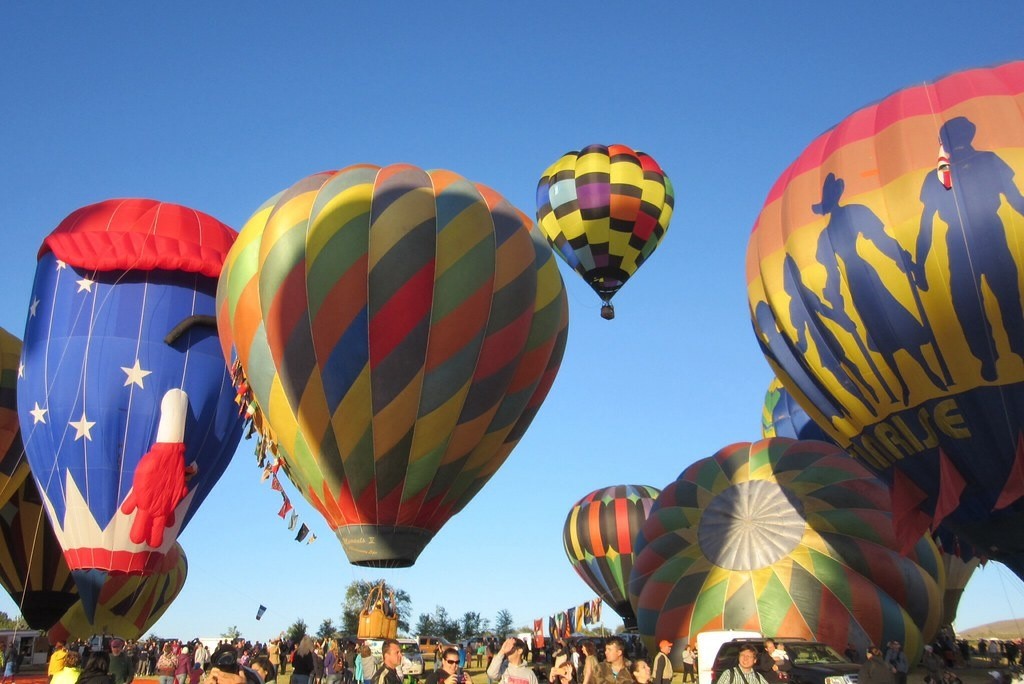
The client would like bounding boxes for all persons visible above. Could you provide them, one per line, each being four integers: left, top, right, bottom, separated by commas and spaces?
47, 634, 1024, 684
0, 640, 17, 684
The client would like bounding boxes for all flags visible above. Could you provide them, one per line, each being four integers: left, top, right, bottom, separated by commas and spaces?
534, 598, 602, 649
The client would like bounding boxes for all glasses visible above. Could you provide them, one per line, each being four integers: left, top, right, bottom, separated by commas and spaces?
444, 658, 461, 664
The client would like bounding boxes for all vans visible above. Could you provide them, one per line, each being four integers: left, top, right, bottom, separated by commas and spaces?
459, 637, 499, 655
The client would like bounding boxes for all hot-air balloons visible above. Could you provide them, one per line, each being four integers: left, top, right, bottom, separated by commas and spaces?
0, 471, 80, 653
745, 61, 1024, 579
48, 540, 188, 651
15, 200, 249, 669
629, 436, 947, 673
0, 328, 32, 508
563, 484, 663, 640
763, 376, 981, 642
533, 143, 675, 320
215, 162, 570, 639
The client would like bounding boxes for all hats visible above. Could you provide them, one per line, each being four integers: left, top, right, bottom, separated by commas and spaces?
925, 645, 933, 651
659, 640, 674, 648
988, 670, 1001, 680
111, 639, 122, 648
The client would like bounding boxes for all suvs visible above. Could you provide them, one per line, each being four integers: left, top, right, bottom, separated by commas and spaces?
78, 634, 127, 659
417, 636, 459, 660
711, 636, 863, 684
396, 639, 425, 676
